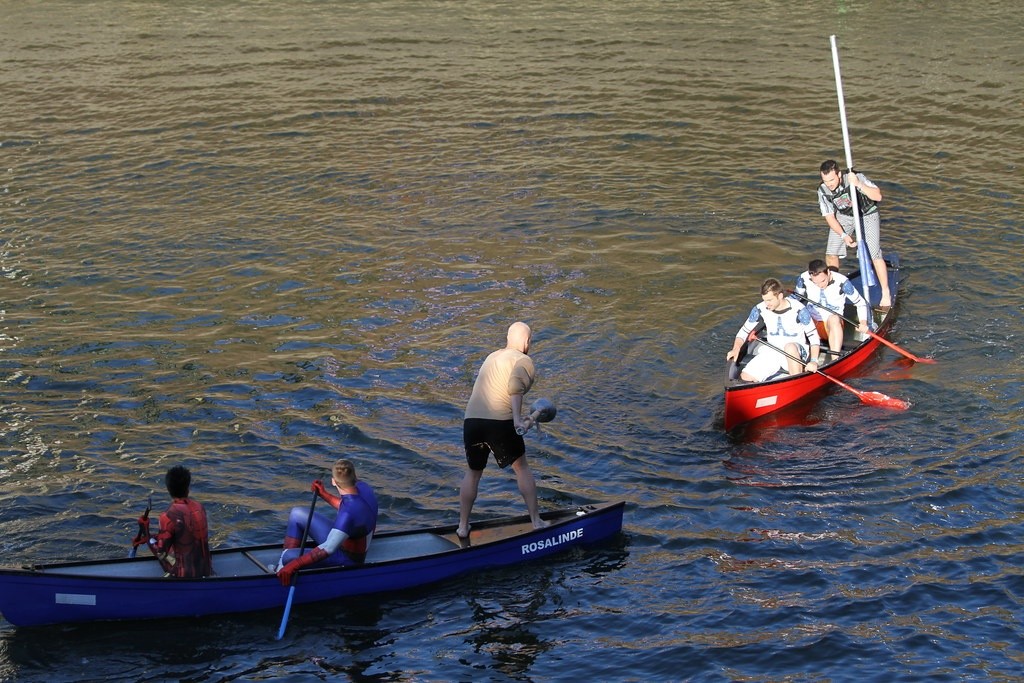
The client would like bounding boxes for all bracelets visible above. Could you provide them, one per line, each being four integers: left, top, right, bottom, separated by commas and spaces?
811, 359, 818, 363
842, 233, 846, 239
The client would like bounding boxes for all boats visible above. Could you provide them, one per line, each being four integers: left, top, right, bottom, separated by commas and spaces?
725, 251, 901, 433
0, 501, 625, 627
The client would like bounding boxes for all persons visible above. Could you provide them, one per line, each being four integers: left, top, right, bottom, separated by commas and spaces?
132, 466, 213, 577
788, 259, 869, 360
277, 459, 378, 586
726, 279, 821, 382
817, 160, 892, 307
456, 322, 550, 539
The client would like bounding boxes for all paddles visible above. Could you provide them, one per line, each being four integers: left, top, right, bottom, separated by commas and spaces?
748, 330, 907, 409
128, 498, 152, 559
828, 32, 878, 333
784, 287, 934, 363
276, 471, 325, 641
